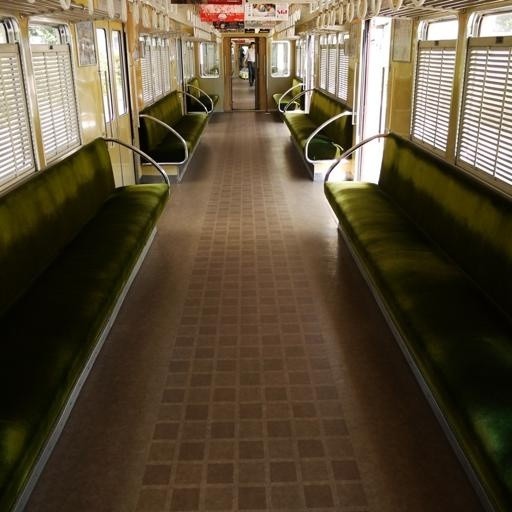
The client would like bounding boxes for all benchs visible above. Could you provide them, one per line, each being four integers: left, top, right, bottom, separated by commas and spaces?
138, 89, 208, 184
272, 77, 304, 121
282, 87, 358, 181
186, 78, 219, 118
0, 137, 170, 512
324, 130, 512, 512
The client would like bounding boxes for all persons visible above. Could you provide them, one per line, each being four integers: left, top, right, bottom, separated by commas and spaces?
242, 41, 256, 86
